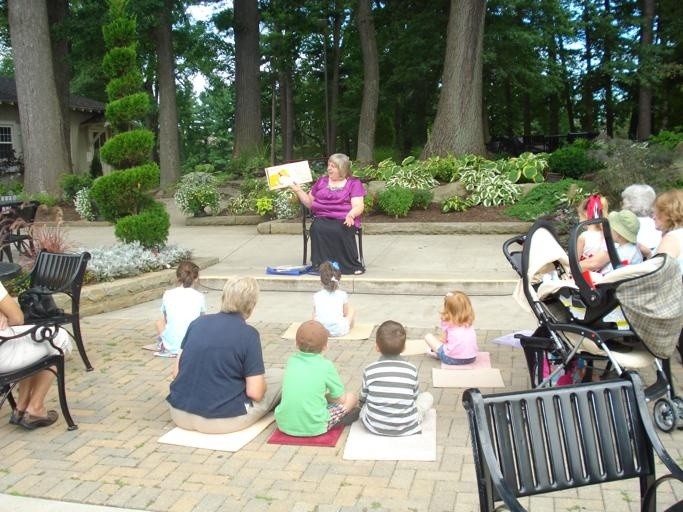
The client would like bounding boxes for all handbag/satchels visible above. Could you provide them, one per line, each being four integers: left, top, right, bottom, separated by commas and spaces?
18, 287, 60, 320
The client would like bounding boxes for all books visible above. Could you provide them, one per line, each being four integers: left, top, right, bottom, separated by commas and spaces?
263, 159, 314, 192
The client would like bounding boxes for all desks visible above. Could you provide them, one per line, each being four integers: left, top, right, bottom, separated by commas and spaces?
0, 261, 21, 281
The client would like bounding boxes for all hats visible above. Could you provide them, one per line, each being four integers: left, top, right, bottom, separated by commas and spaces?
608, 208, 641, 243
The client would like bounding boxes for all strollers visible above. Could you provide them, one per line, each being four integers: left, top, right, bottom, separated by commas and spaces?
504, 219, 683, 433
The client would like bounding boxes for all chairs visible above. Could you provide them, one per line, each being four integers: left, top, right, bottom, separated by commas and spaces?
0, 201, 39, 263
0, 316, 77, 431
18, 248, 94, 372
462, 370, 683, 512
302, 191, 365, 268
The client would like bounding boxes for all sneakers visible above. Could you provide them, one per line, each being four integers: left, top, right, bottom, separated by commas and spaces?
19, 410, 58, 430
8, 406, 23, 425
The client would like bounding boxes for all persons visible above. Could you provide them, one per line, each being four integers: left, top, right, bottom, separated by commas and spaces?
533, 182, 683, 390
311, 260, 355, 337
287, 151, 368, 276
423, 288, 479, 365
153, 260, 207, 358
356, 317, 434, 436
165, 276, 285, 435
272, 319, 360, 438
0, 279, 75, 431
